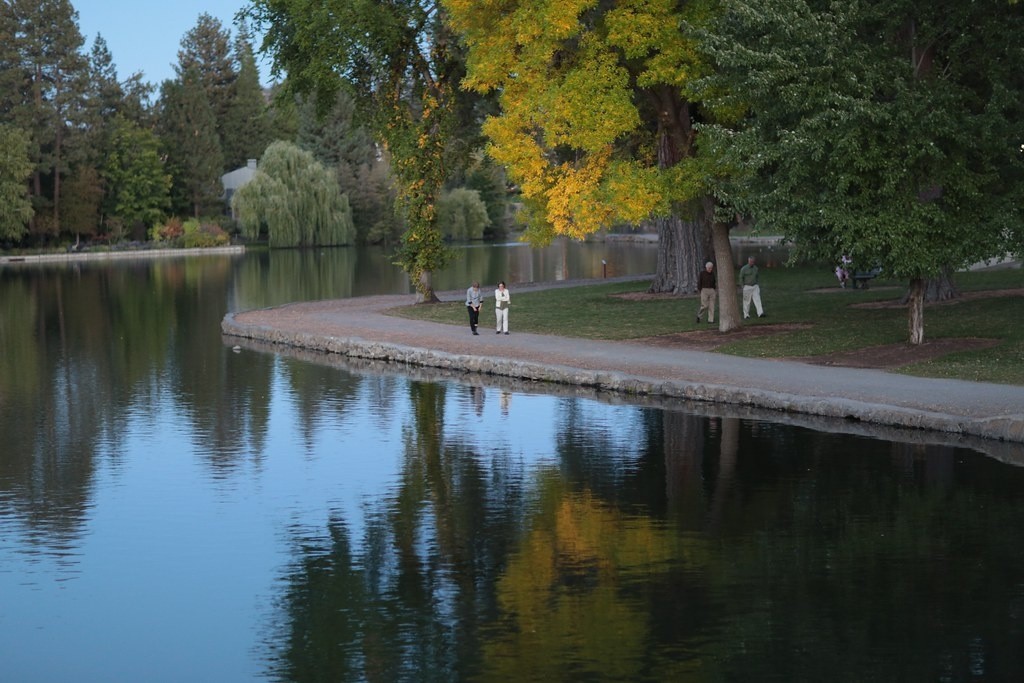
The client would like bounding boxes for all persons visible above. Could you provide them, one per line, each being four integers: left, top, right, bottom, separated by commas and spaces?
739, 256, 765, 319
835, 255, 852, 288
696, 262, 716, 324
465, 282, 484, 335
494, 281, 511, 335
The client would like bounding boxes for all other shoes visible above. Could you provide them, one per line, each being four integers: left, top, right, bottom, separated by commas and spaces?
760, 313, 767, 317
505, 332, 510, 335
472, 331, 478, 335
496, 331, 501, 334
696, 316, 700, 323
707, 322, 716, 324
